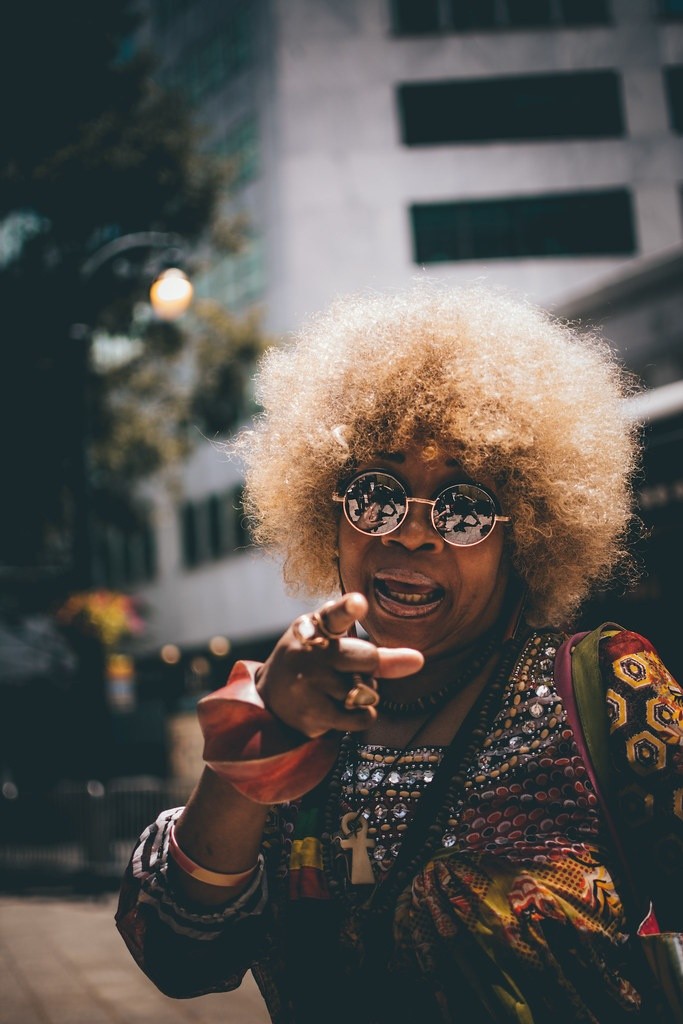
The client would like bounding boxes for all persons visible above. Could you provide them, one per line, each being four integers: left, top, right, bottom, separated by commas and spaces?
113, 271, 683, 1024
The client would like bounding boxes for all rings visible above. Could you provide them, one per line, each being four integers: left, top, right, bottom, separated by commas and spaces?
344, 685, 360, 710
289, 601, 348, 650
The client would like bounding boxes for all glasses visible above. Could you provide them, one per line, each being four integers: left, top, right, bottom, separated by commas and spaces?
332, 466, 511, 547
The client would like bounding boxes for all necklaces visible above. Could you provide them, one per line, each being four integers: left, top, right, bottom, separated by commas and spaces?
322, 620, 528, 924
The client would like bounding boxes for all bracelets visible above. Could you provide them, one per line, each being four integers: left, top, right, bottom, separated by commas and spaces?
169, 824, 258, 887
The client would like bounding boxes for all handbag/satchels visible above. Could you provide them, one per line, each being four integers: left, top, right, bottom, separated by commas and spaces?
555, 622, 683, 1024
